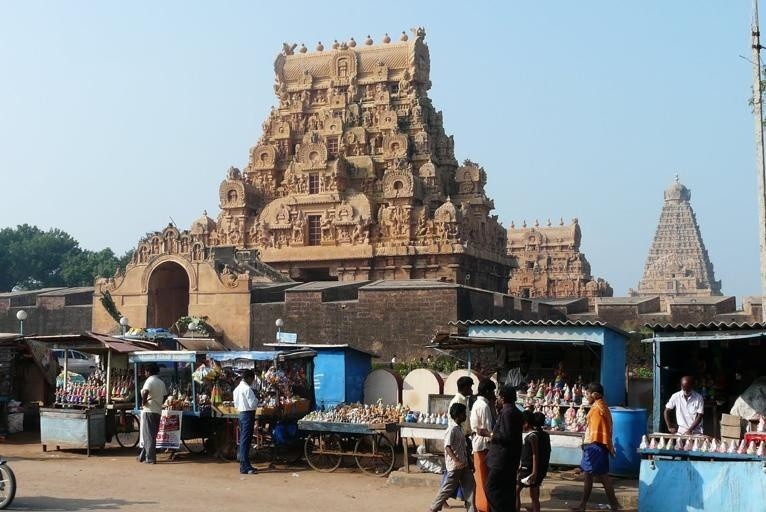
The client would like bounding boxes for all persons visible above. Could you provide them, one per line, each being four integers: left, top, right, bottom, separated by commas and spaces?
552, 377, 589, 431
420, 357, 424, 365
392, 355, 397, 366
568, 384, 621, 512
730, 383, 766, 433
664, 376, 704, 435
429, 377, 552, 511
138, 364, 168, 463
505, 361, 534, 389
428, 355, 431, 362
232, 370, 258, 473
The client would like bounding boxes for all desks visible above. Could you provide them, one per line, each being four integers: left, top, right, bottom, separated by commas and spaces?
396, 421, 447, 473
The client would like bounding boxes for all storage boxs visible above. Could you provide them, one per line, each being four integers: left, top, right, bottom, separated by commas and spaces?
720, 413, 742, 447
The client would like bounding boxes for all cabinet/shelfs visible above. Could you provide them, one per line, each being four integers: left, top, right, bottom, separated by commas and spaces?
523, 403, 589, 467
39, 407, 106, 457
55, 377, 139, 408
636, 448, 766, 512
